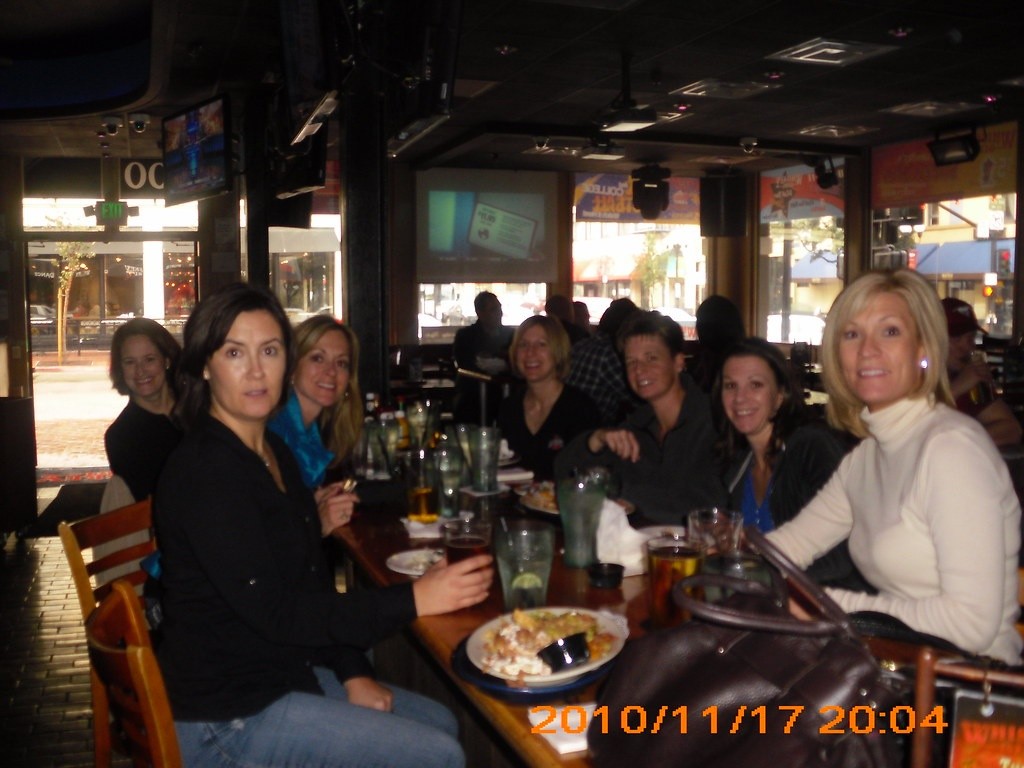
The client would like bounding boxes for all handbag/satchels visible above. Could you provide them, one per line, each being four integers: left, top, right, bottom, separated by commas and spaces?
588, 527, 906, 768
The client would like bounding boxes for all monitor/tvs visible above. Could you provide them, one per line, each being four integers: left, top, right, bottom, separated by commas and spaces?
162, 92, 234, 205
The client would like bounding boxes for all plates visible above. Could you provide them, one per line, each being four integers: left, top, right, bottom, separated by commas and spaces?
498, 456, 523, 469
523, 492, 637, 515
451, 634, 616, 703
385, 548, 444, 577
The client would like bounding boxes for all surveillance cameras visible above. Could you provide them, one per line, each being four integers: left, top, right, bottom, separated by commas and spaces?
740, 137, 758, 154
532, 135, 549, 149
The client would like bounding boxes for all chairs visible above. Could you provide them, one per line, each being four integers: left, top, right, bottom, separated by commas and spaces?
914, 642, 1022, 767
57, 495, 162, 766
82, 577, 188, 768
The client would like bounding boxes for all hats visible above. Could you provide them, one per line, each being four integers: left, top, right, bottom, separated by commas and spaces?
942, 297, 989, 337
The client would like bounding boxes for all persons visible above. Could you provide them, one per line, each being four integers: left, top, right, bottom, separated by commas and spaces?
454, 292, 515, 378
151, 283, 495, 768
765, 267, 1024, 670
105, 317, 183, 503
696, 296, 742, 346
933, 296, 1022, 448
545, 295, 590, 344
567, 298, 647, 424
555, 313, 729, 525
711, 335, 850, 586
495, 314, 595, 469
267, 314, 365, 487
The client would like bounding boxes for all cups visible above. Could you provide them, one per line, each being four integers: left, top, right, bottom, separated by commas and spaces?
964, 350, 996, 406
555, 472, 605, 570
441, 521, 493, 564
689, 506, 742, 604
648, 535, 707, 629
493, 519, 556, 612
467, 426, 501, 491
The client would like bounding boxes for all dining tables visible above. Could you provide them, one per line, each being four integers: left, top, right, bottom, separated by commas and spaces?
324, 466, 964, 766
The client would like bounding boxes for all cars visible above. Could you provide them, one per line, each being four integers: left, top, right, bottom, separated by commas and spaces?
30, 305, 73, 317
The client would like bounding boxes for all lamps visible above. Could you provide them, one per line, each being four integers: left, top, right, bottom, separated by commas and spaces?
629, 153, 671, 218
815, 154, 839, 189
924, 120, 978, 167
598, 107, 658, 132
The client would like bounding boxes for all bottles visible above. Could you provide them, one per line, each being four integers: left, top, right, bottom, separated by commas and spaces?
357, 392, 506, 522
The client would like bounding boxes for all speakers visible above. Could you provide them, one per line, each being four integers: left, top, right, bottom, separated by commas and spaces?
700, 173, 746, 237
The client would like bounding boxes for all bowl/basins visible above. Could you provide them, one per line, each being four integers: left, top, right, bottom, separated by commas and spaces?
466, 604, 626, 688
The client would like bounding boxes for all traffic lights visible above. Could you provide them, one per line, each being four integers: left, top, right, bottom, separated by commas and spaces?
995, 249, 1010, 276
